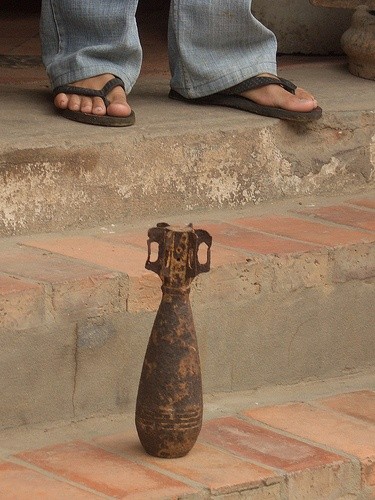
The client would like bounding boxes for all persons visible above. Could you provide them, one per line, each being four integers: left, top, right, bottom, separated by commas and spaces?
38, 0, 323, 123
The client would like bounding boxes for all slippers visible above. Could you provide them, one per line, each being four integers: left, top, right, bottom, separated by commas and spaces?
168, 73, 322, 123
52, 77, 136, 127
